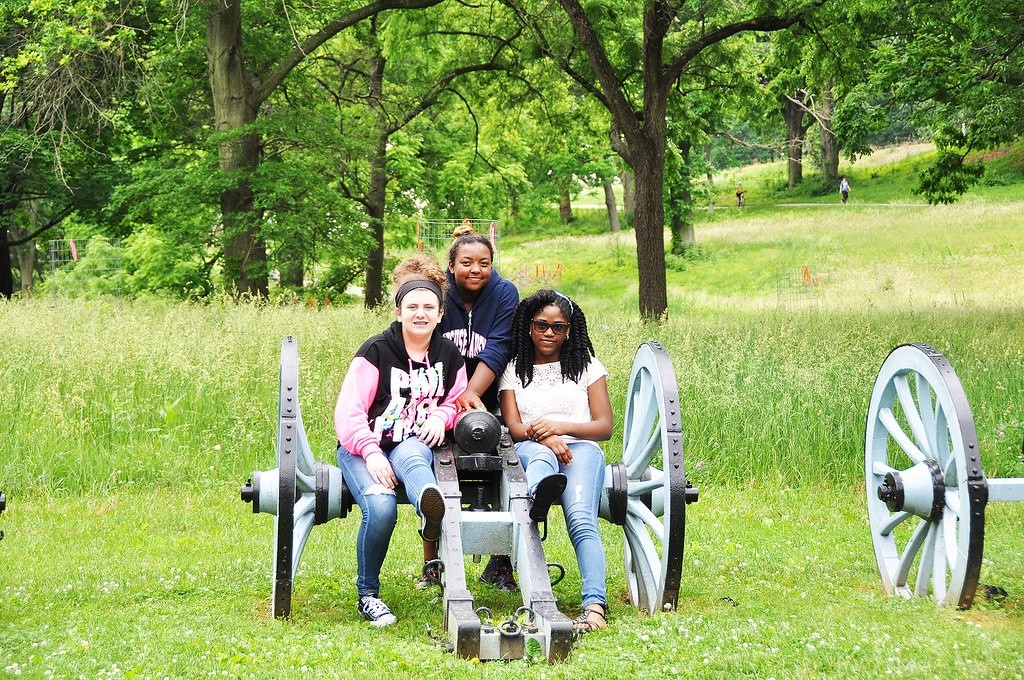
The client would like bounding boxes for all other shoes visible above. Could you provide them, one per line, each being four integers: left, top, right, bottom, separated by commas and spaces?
478, 555, 521, 596
414, 559, 439, 590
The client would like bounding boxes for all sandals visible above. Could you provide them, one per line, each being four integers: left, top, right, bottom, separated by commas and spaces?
529, 472, 568, 522
572, 609, 608, 634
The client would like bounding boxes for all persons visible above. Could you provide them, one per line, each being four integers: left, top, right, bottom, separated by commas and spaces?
840, 175, 851, 204
736, 183, 744, 206
498, 288, 613, 630
418, 233, 520, 593
334, 254, 470, 627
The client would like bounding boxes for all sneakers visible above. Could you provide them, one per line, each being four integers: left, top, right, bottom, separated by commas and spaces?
358, 596, 397, 627
419, 483, 446, 541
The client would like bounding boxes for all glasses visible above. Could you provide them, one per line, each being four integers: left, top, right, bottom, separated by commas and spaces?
530, 318, 569, 335
